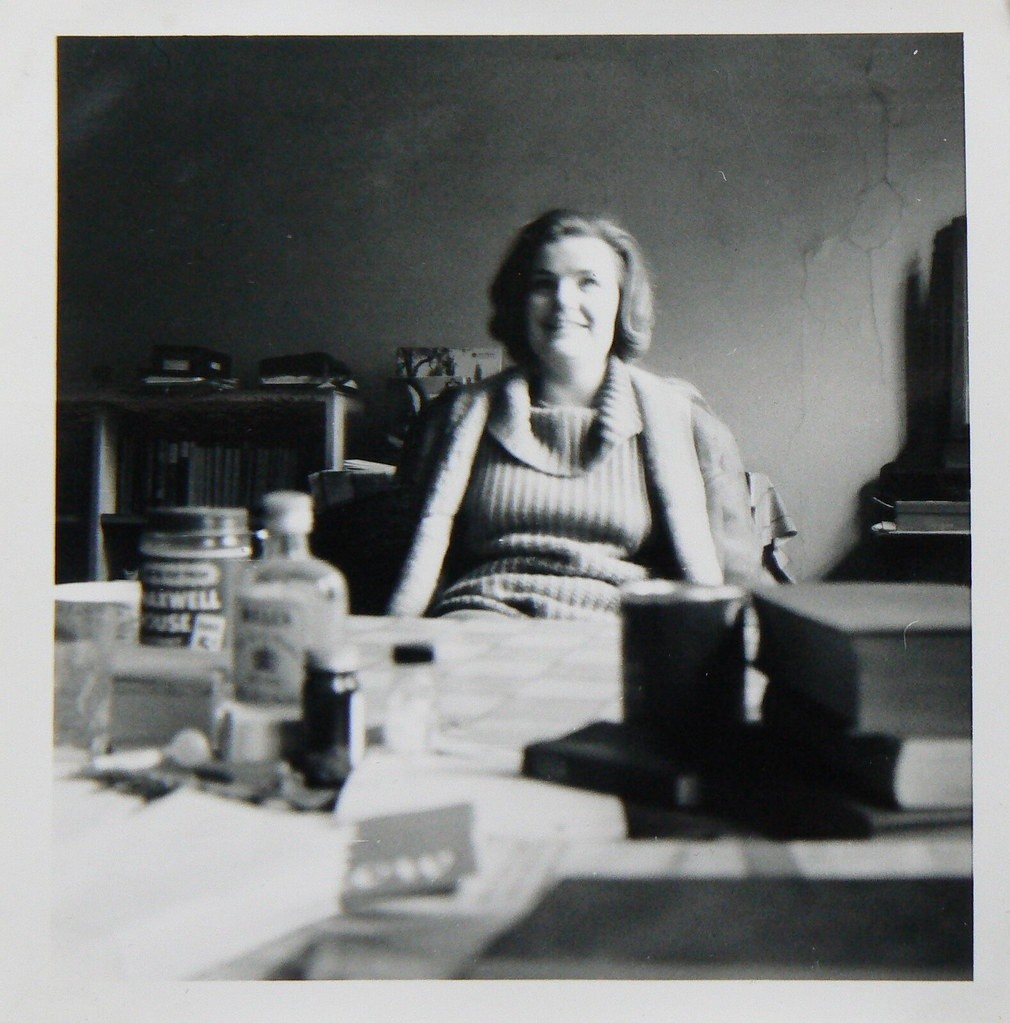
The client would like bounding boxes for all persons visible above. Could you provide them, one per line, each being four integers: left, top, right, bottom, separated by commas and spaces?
360, 211, 790, 624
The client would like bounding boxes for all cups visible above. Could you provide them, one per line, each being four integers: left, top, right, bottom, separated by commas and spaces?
620, 580, 751, 735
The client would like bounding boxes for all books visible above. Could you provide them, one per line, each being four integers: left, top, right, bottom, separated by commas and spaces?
117, 428, 316, 510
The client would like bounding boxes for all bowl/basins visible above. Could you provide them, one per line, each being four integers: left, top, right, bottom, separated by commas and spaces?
54, 580, 142, 648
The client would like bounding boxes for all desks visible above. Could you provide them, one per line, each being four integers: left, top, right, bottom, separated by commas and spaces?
51, 612, 973, 980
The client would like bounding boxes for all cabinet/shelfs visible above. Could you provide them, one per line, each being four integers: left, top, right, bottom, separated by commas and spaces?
53, 389, 383, 579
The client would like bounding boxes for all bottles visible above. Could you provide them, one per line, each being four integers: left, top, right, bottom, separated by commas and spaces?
232, 490, 349, 703
382, 644, 438, 753
300, 645, 367, 786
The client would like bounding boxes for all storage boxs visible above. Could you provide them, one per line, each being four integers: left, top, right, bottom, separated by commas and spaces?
895, 499, 970, 531
